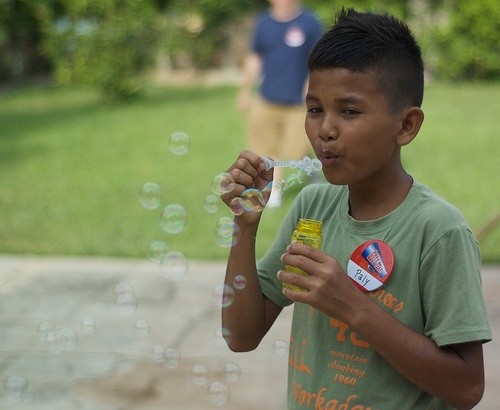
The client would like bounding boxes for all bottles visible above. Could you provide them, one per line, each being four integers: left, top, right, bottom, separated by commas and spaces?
281, 217, 325, 297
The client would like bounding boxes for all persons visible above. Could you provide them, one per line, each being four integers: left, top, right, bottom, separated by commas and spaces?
237, 0, 325, 206
211, 8, 493, 410
